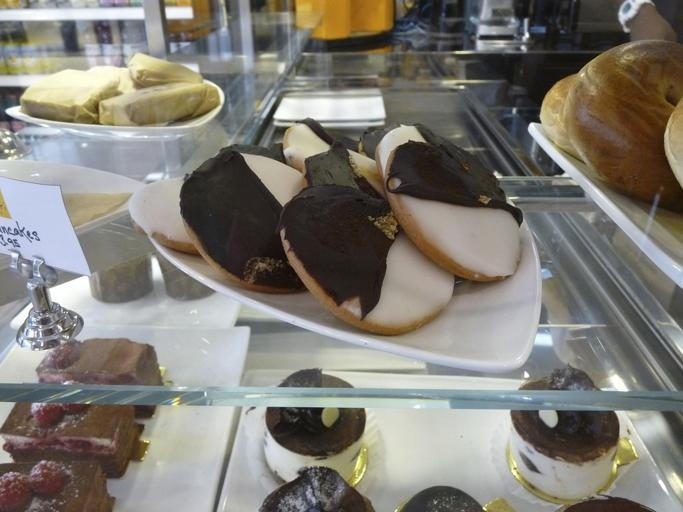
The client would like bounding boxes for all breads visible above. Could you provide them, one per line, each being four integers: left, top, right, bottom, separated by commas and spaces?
283, 123, 386, 199
99, 82, 219, 127
279, 183, 455, 335
127, 176, 200, 255
563, 39, 683, 210
129, 53, 203, 87
377, 125, 521, 283
21, 70, 120, 123
539, 71, 585, 163
180, 151, 307, 295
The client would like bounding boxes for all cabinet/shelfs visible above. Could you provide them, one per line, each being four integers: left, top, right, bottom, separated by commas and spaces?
0, 0, 683, 512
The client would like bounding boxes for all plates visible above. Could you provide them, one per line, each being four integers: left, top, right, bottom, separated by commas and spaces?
4, 79, 225, 140
216, 392, 683, 512
0, 160, 146, 235
273, 88, 387, 128
10, 254, 245, 329
147, 199, 542, 374
0, 325, 250, 511
529, 122, 683, 286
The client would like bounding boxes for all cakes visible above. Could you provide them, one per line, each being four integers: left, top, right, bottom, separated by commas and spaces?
509, 365, 620, 498
0, 461, 116, 512
258, 466, 374, 512
36, 338, 164, 417
392, 486, 483, 511
0, 403, 137, 478
263, 368, 367, 485
